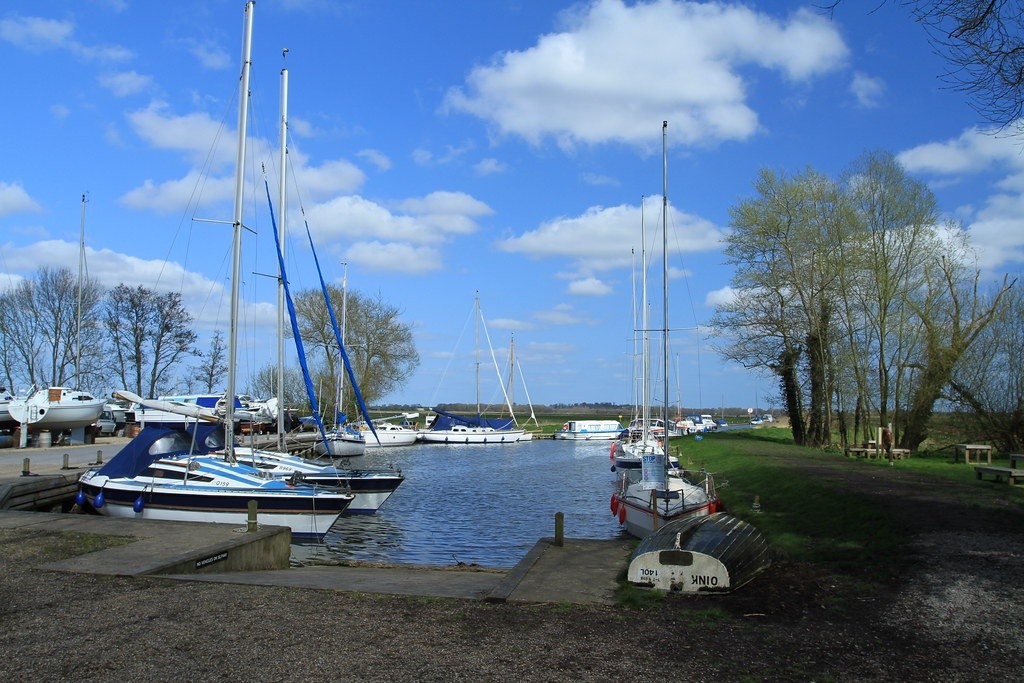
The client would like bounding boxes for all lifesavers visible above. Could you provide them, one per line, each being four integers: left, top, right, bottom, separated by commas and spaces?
562, 424, 568, 431
609, 442, 616, 460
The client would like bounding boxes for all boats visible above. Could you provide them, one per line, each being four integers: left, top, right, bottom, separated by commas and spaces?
416, 289, 554, 444
0, 194, 105, 429
748, 413, 774, 425
315, 260, 367, 456
554, 419, 625, 440
76, 0, 404, 541
358, 413, 419, 447
135, 391, 280, 426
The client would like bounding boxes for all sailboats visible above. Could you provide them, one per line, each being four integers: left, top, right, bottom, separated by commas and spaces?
609, 120, 718, 540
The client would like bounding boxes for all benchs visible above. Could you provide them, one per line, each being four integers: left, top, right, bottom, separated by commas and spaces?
844, 447, 910, 460
973, 466, 1024, 486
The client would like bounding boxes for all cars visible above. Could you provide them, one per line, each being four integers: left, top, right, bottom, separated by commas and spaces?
94, 411, 116, 435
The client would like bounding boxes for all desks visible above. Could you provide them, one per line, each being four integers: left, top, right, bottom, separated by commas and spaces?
1009, 454, 1024, 470
954, 443, 992, 465
861, 440, 893, 460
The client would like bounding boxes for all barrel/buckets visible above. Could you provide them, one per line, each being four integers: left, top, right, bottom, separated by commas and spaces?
31, 434, 39, 447
39, 433, 51, 447
